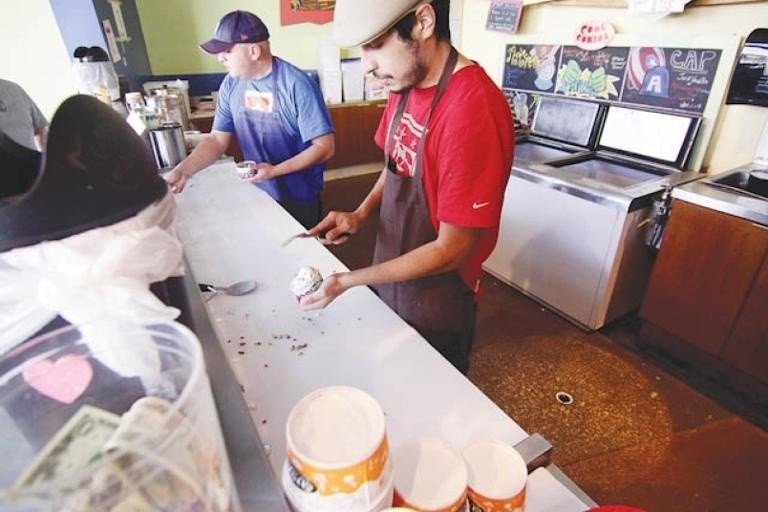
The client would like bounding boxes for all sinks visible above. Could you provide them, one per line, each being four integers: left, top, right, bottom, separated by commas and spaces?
711, 167, 767, 199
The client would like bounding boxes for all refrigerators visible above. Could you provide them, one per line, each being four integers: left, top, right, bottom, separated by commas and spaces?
476, 135, 682, 334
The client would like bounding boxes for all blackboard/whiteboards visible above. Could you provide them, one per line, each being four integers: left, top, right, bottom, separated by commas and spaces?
500, 45, 721, 118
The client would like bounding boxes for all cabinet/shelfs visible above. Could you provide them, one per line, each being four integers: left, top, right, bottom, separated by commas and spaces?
636, 198, 768, 406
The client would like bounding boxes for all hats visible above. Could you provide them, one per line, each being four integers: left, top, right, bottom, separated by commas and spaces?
199, 11, 269, 56
333, 0, 433, 50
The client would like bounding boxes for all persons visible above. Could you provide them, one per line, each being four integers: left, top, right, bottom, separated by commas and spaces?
0, 80, 50, 198
162, 10, 336, 239
289, 0, 516, 376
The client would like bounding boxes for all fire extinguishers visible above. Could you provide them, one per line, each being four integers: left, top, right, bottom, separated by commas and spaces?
638, 183, 672, 251
